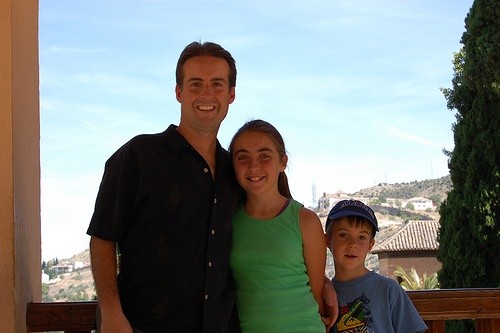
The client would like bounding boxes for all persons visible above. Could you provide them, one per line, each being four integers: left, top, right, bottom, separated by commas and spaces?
87, 41, 339, 333
324, 200, 430, 333
226, 119, 327, 333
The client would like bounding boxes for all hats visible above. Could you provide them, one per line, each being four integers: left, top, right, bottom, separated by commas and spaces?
325, 200, 379, 239
